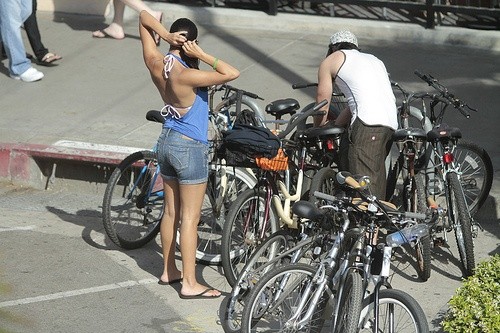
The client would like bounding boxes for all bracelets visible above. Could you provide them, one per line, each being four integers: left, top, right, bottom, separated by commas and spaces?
213, 57, 219, 70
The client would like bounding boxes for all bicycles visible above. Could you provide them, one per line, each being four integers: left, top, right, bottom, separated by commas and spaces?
389, 80, 452, 281
224, 170, 431, 333
263, 82, 318, 136
413, 68, 493, 277
221, 99, 329, 289
101, 81, 265, 250
308, 91, 350, 214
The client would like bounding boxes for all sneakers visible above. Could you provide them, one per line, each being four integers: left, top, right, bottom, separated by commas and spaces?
9, 67, 44, 82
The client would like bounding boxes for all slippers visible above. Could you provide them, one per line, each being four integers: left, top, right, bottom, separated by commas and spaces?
180, 288, 222, 299
158, 279, 181, 285
36, 53, 62, 65
152, 12, 162, 46
93, 25, 125, 39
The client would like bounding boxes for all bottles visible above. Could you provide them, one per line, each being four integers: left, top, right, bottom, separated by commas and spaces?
385, 224, 430, 245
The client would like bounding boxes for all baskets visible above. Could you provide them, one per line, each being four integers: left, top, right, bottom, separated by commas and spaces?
205, 137, 290, 171
330, 92, 349, 112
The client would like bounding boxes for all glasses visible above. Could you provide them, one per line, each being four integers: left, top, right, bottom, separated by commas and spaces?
195, 40, 199, 45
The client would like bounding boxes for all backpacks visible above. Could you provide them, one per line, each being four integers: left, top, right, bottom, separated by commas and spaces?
218, 108, 280, 163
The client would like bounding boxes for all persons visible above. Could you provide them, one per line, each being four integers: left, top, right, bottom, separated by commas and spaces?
0, 0, 63, 82
138, 11, 240, 299
313, 30, 398, 200
92, 0, 164, 46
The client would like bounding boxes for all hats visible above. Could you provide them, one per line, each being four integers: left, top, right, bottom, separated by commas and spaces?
325, 31, 358, 57
